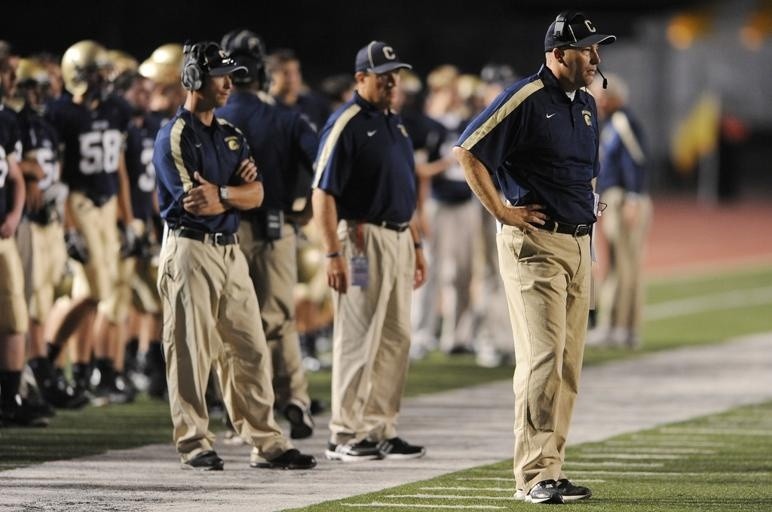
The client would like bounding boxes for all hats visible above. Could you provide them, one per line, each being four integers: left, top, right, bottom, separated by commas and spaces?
354, 39, 412, 74
544, 16, 616, 52
182, 40, 249, 75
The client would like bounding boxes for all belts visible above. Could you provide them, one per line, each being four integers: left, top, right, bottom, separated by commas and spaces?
375, 221, 408, 233
176, 228, 239, 246
534, 221, 592, 237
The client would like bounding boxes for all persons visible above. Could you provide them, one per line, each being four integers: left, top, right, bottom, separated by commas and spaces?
153, 40, 320, 473
219, 30, 319, 440
450, 11, 621, 505
0, 41, 648, 426
311, 36, 431, 464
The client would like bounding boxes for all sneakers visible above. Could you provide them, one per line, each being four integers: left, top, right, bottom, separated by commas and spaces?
515, 479, 592, 501
283, 398, 313, 438
526, 480, 564, 504
376, 438, 425, 458
250, 448, 316, 469
1, 359, 167, 427
325, 438, 386, 462
180, 450, 224, 470
223, 430, 244, 445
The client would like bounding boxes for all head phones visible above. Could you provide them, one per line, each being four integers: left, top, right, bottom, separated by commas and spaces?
180, 39, 203, 91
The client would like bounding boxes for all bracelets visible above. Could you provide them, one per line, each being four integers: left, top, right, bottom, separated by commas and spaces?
219, 183, 231, 209
325, 251, 346, 260
413, 240, 424, 250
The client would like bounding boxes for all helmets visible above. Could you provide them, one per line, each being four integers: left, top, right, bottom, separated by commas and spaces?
11, 41, 187, 98
221, 30, 272, 93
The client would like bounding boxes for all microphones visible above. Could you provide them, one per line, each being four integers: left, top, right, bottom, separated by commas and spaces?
597, 67, 607, 89
